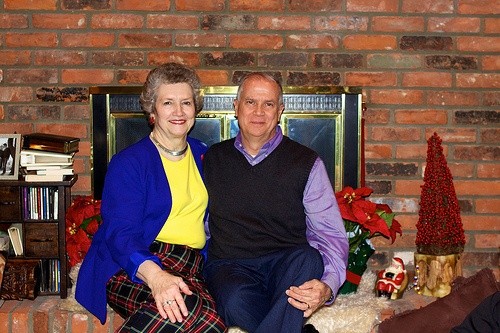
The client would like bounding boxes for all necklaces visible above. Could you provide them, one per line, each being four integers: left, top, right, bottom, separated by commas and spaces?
151, 132, 188, 156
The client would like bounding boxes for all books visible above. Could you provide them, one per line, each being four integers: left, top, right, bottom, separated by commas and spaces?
0, 132, 80, 301
22, 187, 59, 221
7, 222, 24, 257
38, 260, 60, 293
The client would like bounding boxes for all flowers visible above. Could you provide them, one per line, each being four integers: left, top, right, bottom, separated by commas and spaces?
334, 182, 402, 294
64, 195, 103, 271
413, 131, 466, 255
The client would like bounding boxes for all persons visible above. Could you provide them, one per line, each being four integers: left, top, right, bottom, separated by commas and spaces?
202, 72, 350, 333
1, 143, 10, 175
0, 149, 4, 170
73, 62, 229, 333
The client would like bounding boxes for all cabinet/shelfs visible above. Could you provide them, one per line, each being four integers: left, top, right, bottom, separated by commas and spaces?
0, 180, 72, 301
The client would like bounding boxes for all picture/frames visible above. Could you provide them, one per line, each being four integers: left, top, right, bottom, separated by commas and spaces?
0, 133, 21, 179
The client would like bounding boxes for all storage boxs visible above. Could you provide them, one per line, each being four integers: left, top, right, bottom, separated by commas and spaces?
25, 223, 59, 258
0, 186, 22, 221
0, 258, 42, 300
22, 133, 80, 154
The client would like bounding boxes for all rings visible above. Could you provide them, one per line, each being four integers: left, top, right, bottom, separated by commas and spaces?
307, 304, 310, 309
163, 300, 176, 307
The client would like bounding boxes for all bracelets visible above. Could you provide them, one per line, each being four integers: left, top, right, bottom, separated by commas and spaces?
325, 290, 333, 304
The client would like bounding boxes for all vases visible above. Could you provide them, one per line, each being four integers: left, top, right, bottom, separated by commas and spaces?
413, 251, 464, 298
